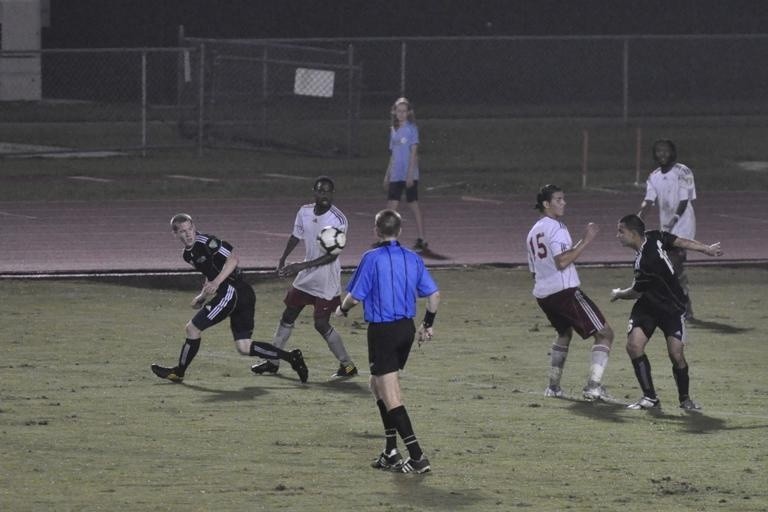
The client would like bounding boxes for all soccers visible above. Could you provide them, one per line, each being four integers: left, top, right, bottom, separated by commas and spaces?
317, 225, 346, 255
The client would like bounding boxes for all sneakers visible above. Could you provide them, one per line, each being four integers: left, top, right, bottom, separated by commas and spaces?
581, 386, 625, 404
331, 359, 357, 383
289, 350, 308, 382
399, 453, 431, 474
371, 449, 403, 472
628, 397, 660, 409
250, 361, 277, 375
151, 363, 184, 384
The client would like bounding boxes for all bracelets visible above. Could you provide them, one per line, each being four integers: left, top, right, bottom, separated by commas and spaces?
423, 310, 436, 329
340, 305, 349, 313
673, 214, 680, 220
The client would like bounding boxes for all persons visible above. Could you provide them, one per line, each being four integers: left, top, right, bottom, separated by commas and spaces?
335, 210, 441, 475
250, 177, 358, 382
636, 138, 697, 327
150, 213, 308, 385
526, 185, 616, 402
372, 98, 429, 254
610, 215, 725, 412
